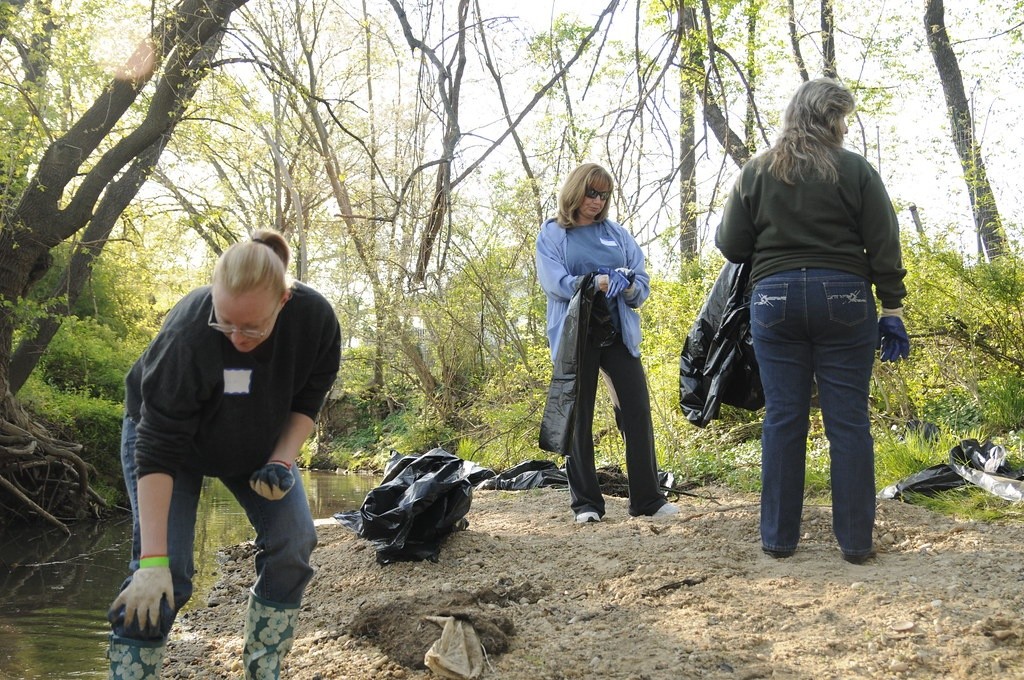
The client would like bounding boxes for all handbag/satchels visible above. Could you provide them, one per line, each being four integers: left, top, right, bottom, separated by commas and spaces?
720, 342, 766, 412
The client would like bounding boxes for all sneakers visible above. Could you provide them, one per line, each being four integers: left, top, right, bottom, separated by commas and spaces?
652, 503, 680, 517
576, 511, 601, 524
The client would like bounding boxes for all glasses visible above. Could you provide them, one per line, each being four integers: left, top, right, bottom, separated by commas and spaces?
207, 295, 281, 339
585, 185, 611, 200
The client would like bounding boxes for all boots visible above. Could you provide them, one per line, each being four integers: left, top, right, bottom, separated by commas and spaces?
241, 585, 302, 680
106, 635, 167, 680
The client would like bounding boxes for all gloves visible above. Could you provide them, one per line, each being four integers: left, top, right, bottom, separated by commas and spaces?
597, 266, 636, 299
875, 308, 910, 363
249, 460, 296, 501
109, 553, 176, 630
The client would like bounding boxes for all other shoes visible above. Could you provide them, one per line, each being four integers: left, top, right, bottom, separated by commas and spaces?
761, 545, 795, 559
843, 552, 870, 565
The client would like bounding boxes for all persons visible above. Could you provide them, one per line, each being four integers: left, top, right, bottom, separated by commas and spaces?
713, 75, 911, 564
535, 163, 682, 523
104, 228, 345, 680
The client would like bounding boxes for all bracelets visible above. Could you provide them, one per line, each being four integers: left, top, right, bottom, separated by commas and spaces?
268, 459, 291, 469
139, 554, 171, 567
